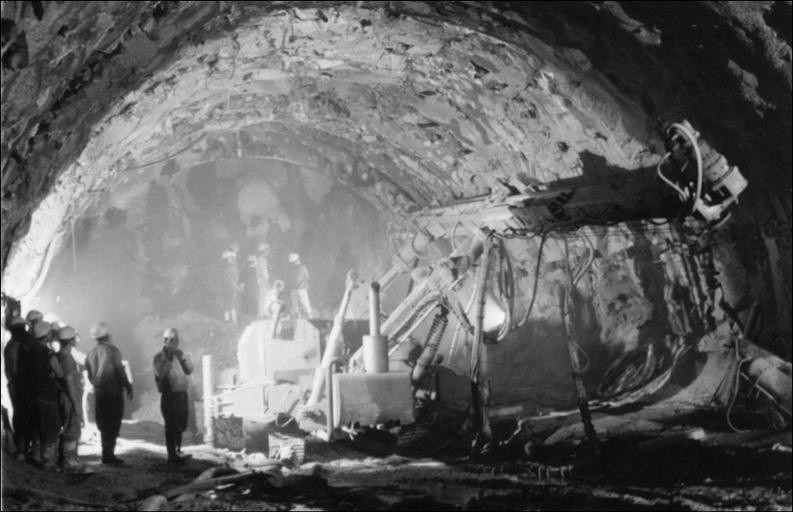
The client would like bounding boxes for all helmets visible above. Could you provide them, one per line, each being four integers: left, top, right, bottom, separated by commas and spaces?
289, 253, 298, 263
9, 311, 76, 340
92, 323, 108, 340
163, 327, 179, 339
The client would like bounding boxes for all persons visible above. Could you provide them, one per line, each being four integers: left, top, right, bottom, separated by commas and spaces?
2, 307, 95, 477
219, 238, 310, 323
152, 325, 195, 468
84, 319, 134, 464
